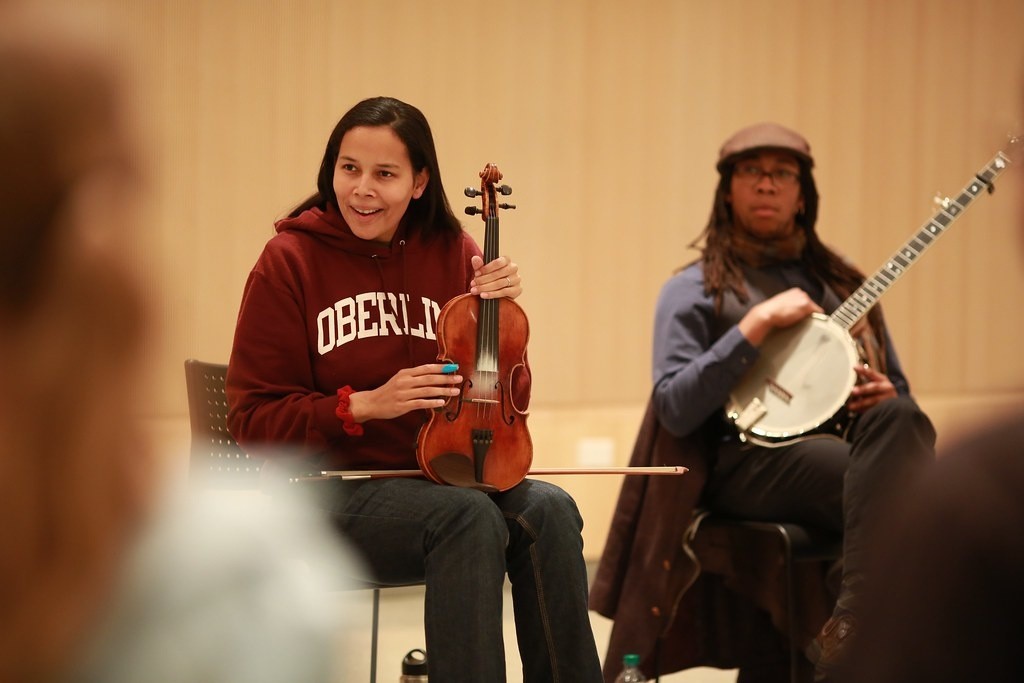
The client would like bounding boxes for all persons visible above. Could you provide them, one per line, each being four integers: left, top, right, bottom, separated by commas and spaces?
834, 399, 1024, 683
227, 96, 607, 683
653, 123, 937, 683
0, 29, 160, 683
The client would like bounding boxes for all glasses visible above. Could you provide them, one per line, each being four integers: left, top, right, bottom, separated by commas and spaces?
734, 164, 800, 186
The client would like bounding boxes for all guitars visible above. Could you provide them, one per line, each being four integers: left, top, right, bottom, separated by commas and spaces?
723, 128, 1024, 447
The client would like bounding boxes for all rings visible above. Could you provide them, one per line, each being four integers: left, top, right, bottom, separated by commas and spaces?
505, 277, 511, 287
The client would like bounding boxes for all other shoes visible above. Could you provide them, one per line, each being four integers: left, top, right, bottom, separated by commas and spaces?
806, 616, 858, 674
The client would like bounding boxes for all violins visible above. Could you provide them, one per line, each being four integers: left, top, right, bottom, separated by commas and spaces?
412, 160, 533, 495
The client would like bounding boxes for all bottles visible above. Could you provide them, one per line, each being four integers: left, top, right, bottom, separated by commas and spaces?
400, 648, 433, 683
613, 653, 647, 683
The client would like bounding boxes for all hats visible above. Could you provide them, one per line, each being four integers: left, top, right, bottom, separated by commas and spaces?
716, 123, 815, 174
